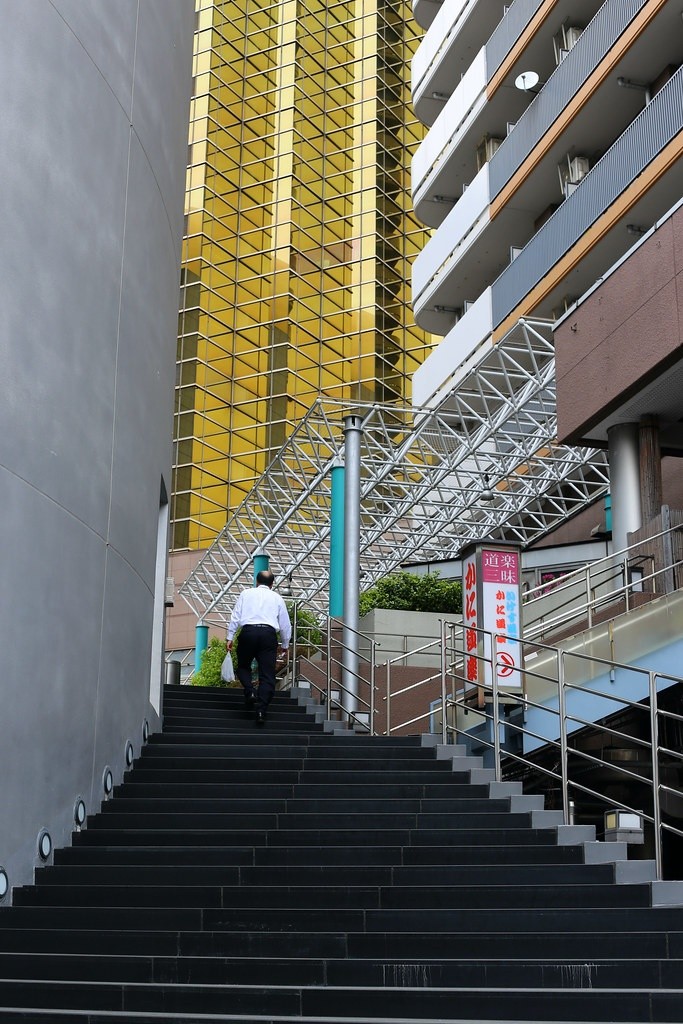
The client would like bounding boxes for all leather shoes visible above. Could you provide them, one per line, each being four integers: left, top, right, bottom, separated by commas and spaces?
257, 710, 266, 723
246, 691, 257, 702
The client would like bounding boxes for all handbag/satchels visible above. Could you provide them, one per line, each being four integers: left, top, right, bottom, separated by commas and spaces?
220, 649, 236, 683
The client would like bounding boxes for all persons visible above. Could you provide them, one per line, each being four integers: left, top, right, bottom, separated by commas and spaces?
225, 571, 292, 726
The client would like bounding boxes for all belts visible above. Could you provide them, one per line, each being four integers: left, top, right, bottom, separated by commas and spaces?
253, 625, 268, 628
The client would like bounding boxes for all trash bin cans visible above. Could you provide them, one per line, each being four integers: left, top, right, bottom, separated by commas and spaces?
165, 659, 181, 685
